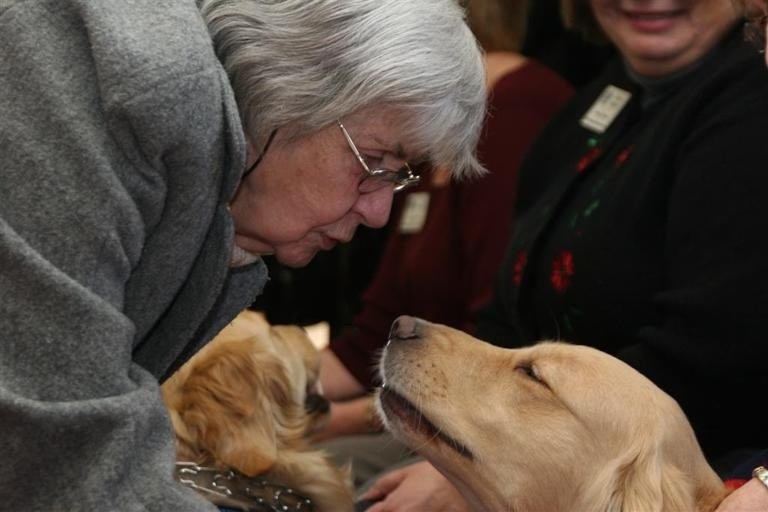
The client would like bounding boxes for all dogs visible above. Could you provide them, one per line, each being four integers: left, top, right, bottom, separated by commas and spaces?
370, 315, 733, 512
159, 308, 362, 512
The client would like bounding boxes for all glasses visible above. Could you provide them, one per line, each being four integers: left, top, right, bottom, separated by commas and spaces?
334, 113, 422, 195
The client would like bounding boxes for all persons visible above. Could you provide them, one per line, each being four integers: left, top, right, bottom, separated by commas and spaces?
714, 460, 767, 511
0, 0, 488, 511
314, 0, 768, 511
269, 1, 580, 403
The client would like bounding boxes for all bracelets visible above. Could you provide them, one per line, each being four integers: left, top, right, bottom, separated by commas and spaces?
754, 465, 768, 491
315, 377, 327, 403
